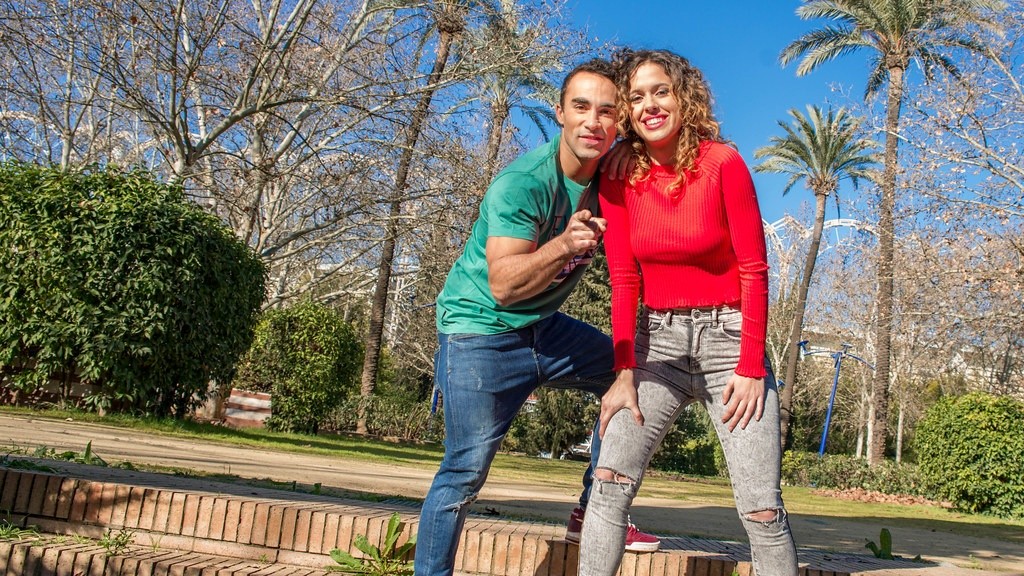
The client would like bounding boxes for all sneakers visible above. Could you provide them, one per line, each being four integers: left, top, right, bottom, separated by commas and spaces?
565, 508, 660, 552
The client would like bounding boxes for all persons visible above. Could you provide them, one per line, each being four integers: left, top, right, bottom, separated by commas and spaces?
414, 57, 660, 576
576, 49, 798, 576
